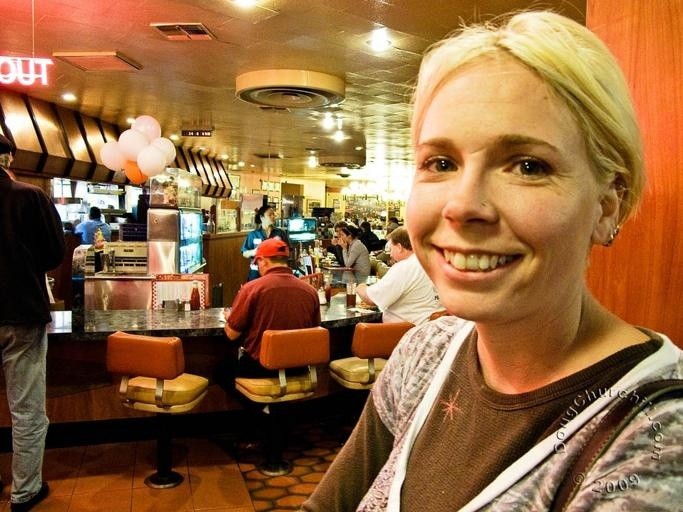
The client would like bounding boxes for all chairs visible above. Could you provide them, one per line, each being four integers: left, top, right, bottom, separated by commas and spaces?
330, 321, 422, 449
105, 327, 210, 492
236, 325, 329, 477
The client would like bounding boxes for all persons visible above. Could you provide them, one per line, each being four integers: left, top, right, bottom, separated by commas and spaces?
0, 133, 63, 512
73, 206, 111, 245
212, 238, 320, 448
241, 205, 293, 281
299, 5, 683, 512
324, 211, 446, 325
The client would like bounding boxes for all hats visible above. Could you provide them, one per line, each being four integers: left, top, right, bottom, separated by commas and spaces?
0, 134, 16, 155
252, 239, 290, 262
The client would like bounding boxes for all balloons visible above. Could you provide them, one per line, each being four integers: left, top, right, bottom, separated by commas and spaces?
100, 114, 177, 185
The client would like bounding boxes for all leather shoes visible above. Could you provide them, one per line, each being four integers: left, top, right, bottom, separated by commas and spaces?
11, 481, 50, 512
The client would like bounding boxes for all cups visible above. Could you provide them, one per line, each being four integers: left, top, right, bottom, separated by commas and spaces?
368, 274, 378, 285
346, 282, 358, 309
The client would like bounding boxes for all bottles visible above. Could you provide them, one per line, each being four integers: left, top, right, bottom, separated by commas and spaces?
323, 275, 332, 304
190, 281, 200, 311
